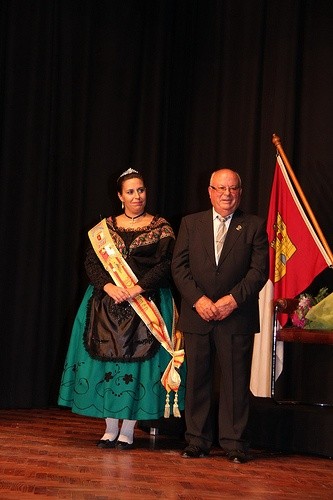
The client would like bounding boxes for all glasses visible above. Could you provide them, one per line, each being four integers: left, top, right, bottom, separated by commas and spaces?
210, 186, 239, 193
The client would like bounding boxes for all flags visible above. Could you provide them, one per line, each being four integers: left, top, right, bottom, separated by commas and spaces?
250, 153, 331, 397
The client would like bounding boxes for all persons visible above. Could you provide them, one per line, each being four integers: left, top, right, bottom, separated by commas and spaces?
169, 168, 269, 465
56, 168, 176, 450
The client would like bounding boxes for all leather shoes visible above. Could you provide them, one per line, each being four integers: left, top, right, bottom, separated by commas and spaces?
225, 448, 248, 464
96, 430, 120, 448
111, 433, 135, 450
182, 444, 209, 458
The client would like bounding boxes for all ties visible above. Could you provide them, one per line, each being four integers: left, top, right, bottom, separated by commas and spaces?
215, 218, 228, 261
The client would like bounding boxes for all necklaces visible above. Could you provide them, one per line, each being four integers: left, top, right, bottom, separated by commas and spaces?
124, 212, 144, 227
